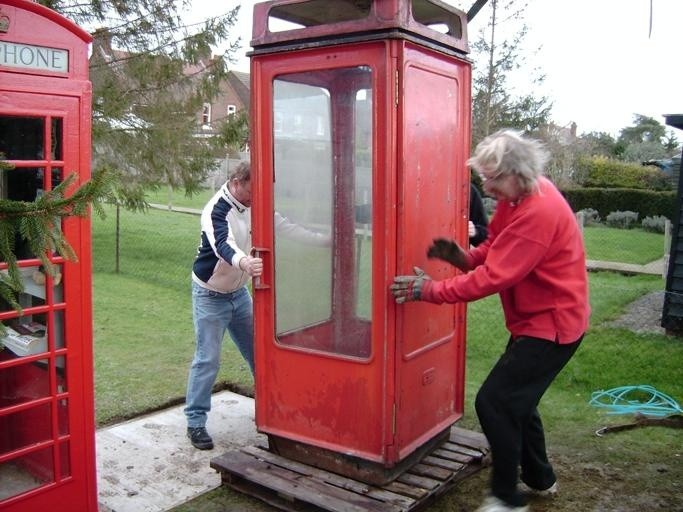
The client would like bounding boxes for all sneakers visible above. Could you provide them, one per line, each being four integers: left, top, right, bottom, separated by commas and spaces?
516, 480, 559, 497
473, 494, 529, 512
185, 426, 214, 450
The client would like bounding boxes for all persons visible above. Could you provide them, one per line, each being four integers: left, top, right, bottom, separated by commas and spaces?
389, 127, 590, 511
181, 158, 334, 452
323, 157, 490, 251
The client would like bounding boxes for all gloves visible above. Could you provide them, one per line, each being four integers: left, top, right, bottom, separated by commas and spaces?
425, 235, 468, 272
388, 267, 432, 305
